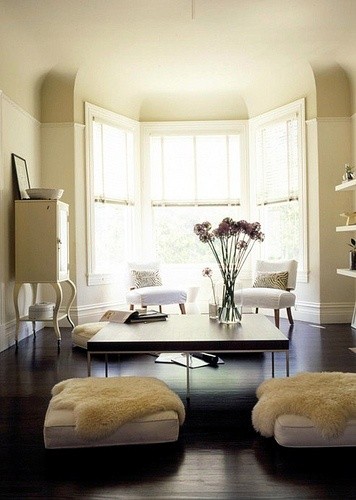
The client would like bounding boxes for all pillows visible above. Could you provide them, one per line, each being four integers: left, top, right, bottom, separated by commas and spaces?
253, 270, 288, 291
131, 268, 161, 289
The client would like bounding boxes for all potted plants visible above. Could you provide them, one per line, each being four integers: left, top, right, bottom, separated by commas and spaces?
344, 238, 356, 270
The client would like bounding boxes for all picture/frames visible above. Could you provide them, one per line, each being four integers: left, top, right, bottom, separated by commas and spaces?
11, 153, 30, 200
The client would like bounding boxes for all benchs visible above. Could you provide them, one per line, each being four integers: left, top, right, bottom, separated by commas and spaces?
70, 321, 107, 350
43, 376, 185, 449
250, 372, 356, 448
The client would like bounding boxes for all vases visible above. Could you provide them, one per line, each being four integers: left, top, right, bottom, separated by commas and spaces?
217, 282, 243, 324
208, 296, 220, 319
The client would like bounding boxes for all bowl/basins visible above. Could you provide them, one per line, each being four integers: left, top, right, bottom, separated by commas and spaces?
25, 188, 64, 200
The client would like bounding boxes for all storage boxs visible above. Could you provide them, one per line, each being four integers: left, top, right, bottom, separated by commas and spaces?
29, 301, 56, 318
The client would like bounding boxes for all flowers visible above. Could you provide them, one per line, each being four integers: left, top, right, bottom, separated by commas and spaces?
193, 217, 265, 322
202, 267, 218, 315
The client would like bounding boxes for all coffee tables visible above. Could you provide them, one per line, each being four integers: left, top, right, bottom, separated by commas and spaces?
86, 314, 290, 399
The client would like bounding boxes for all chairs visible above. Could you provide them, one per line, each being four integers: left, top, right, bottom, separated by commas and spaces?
127, 262, 187, 314
234, 260, 299, 328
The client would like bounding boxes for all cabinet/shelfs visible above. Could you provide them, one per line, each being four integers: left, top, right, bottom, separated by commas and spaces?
335, 175, 356, 279
14, 200, 77, 349
